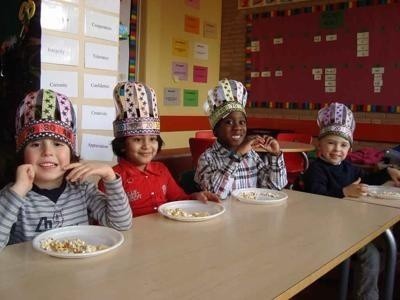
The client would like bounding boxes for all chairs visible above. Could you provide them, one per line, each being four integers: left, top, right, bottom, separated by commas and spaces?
195, 131, 216, 137
189, 137, 216, 171
276, 133, 314, 186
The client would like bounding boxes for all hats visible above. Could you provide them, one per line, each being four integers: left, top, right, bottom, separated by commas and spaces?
15, 90, 75, 157
113, 82, 160, 138
316, 102, 356, 148
203, 80, 248, 132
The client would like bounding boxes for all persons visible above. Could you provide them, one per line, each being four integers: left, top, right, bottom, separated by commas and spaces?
303, 119, 400, 300
0, 106, 133, 250
94, 109, 221, 224
194, 97, 289, 200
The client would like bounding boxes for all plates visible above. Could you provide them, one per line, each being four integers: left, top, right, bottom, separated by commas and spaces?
34, 224, 124, 260
368, 186, 400, 200
157, 200, 226, 222
230, 188, 288, 205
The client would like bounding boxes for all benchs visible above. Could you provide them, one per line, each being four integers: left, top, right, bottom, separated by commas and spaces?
153, 153, 208, 200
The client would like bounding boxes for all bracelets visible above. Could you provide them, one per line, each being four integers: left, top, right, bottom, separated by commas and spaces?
229, 156, 241, 163
